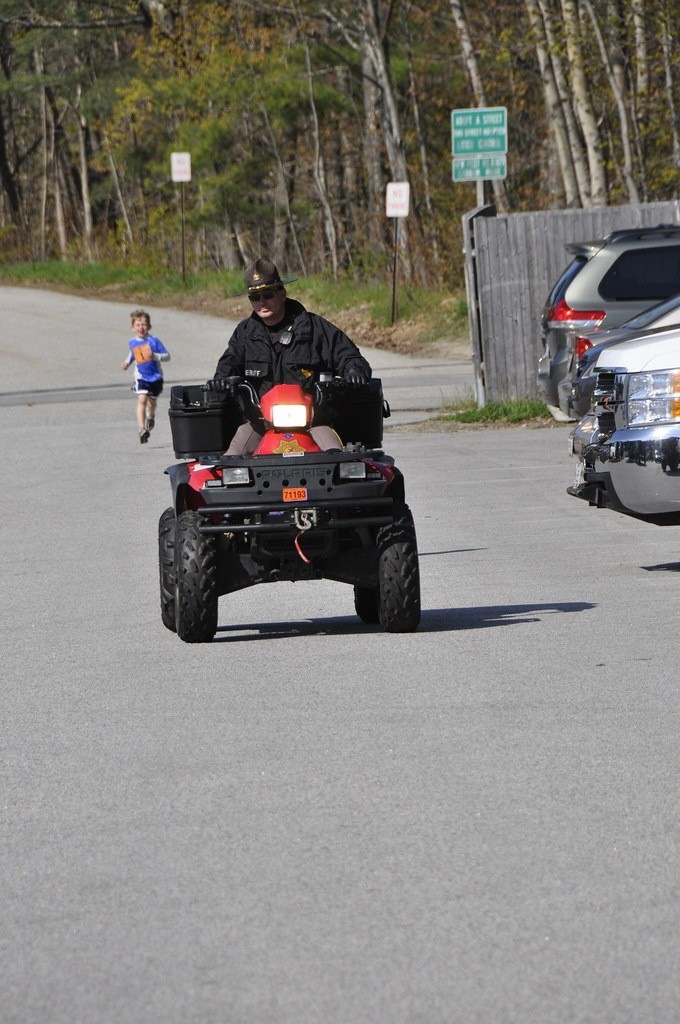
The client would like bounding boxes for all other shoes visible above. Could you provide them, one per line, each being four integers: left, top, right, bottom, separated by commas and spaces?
138, 429, 150, 443
145, 418, 155, 431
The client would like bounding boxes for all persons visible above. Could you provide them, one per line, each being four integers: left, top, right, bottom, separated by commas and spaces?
119, 308, 171, 445
200, 256, 374, 520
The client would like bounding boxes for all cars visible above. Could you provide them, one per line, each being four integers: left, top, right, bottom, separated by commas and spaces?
556, 285, 680, 521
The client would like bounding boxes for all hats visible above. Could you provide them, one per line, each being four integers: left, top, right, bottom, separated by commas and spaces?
233, 258, 298, 296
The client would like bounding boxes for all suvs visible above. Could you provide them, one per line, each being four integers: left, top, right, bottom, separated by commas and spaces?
533, 220, 680, 423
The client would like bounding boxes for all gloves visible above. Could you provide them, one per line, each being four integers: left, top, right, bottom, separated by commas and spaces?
207, 376, 230, 396
344, 368, 367, 389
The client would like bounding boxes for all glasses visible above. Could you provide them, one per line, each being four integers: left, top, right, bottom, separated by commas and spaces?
248, 289, 279, 301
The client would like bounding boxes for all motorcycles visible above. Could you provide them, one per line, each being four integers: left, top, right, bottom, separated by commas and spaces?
157, 371, 420, 643
